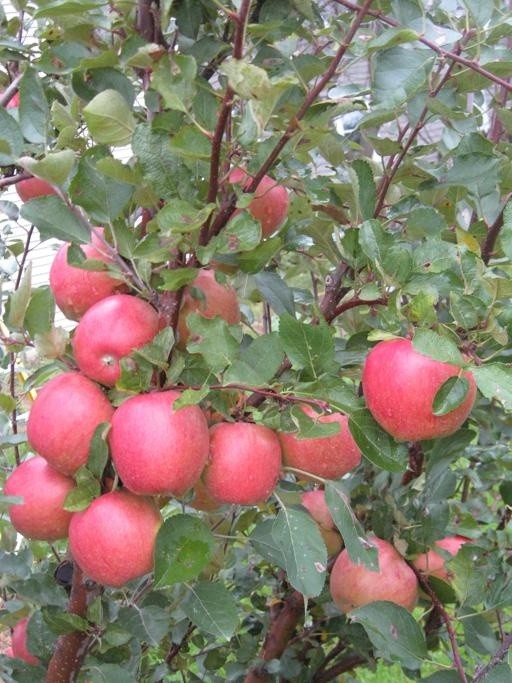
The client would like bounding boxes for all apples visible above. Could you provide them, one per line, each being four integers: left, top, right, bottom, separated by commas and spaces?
4, 90, 478, 668
